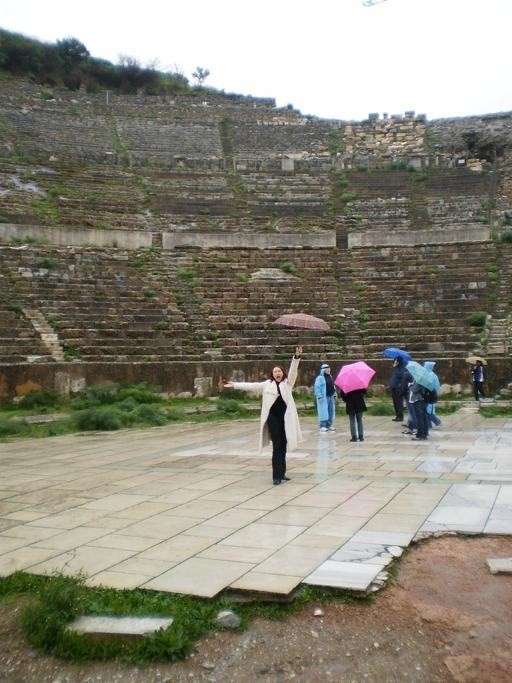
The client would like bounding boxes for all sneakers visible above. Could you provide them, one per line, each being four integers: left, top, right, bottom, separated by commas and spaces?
321, 426, 336, 432
401, 422, 443, 440
350, 436, 364, 442
392, 417, 403, 421
273, 476, 290, 485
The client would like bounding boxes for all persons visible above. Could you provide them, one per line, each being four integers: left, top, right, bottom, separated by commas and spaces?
314, 363, 338, 432
389, 356, 405, 422
340, 388, 368, 442
408, 378, 429, 441
471, 360, 487, 401
424, 361, 442, 427
401, 370, 418, 435
223, 346, 303, 485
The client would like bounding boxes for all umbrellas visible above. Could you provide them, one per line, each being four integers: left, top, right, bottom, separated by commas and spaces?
273, 312, 330, 350
334, 360, 376, 394
465, 356, 488, 366
405, 360, 434, 392
383, 347, 412, 362
